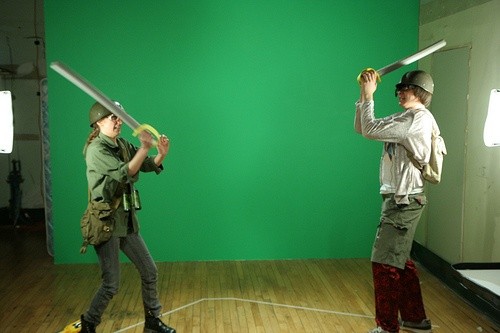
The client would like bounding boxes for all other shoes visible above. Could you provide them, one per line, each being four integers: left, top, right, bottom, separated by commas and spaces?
367, 326, 389, 333
398, 318, 432, 332
81, 322, 95, 333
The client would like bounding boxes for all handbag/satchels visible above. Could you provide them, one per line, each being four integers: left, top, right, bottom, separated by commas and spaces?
80, 201, 115, 254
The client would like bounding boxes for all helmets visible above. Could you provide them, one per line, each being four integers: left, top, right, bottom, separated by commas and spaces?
400, 70, 434, 94
89, 101, 124, 126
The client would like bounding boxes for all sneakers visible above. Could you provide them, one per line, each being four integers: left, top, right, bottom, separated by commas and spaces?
144, 317, 176, 333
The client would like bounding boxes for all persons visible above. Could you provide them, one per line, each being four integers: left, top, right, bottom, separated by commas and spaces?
78, 99, 176, 333
353, 69, 441, 333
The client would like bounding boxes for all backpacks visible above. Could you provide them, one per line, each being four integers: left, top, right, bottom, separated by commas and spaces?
403, 110, 447, 185
